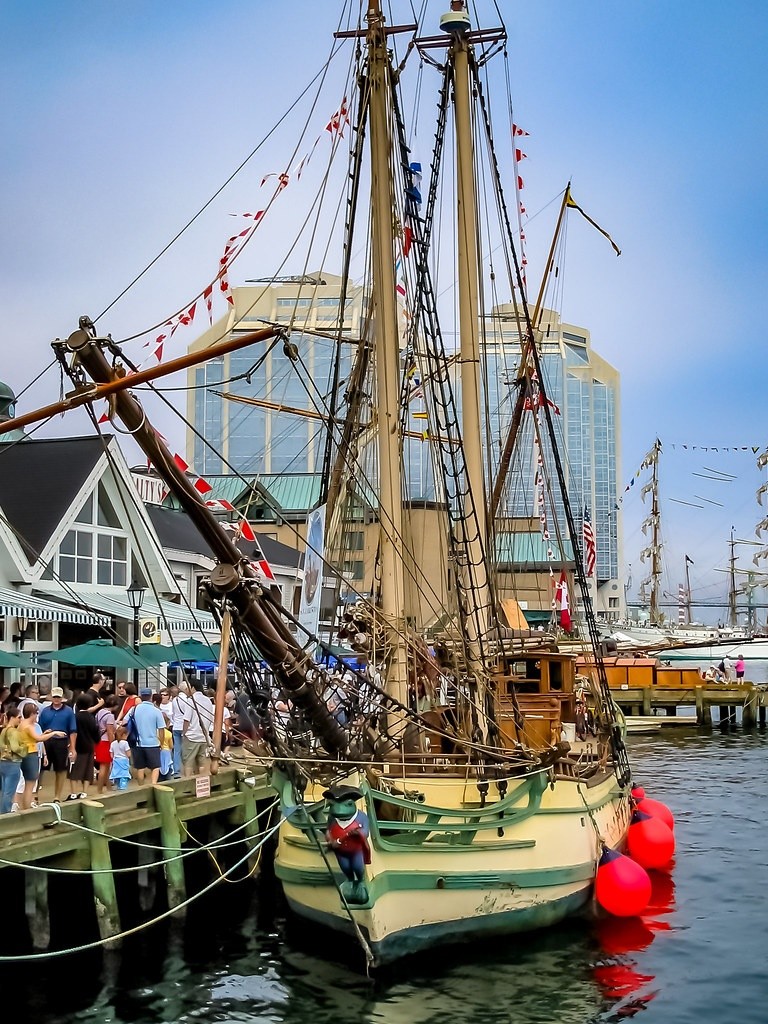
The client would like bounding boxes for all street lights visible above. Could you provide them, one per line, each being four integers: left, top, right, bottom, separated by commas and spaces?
335, 597, 346, 674
124, 579, 147, 697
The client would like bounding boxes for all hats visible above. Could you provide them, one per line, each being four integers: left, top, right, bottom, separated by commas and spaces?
140, 688, 152, 695
51, 687, 63, 698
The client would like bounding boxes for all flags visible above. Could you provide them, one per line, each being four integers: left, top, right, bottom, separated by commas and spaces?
556, 570, 571, 632
584, 508, 596, 576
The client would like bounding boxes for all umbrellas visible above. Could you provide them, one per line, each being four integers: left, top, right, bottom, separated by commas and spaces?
0, 649, 47, 670
31, 639, 154, 672
173, 636, 218, 660
318, 641, 354, 656
129, 644, 196, 664
242, 641, 268, 661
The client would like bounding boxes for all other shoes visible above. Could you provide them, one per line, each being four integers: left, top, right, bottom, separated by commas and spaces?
64, 794, 77, 802
11, 803, 19, 812
31, 802, 38, 808
78, 792, 87, 799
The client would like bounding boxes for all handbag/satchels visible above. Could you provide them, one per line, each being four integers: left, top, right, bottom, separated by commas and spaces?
719, 659, 725, 673
128, 706, 140, 748
1, 726, 24, 761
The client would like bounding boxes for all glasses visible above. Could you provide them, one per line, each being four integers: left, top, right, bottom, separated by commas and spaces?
35, 713, 39, 714
119, 686, 123, 689
161, 694, 168, 696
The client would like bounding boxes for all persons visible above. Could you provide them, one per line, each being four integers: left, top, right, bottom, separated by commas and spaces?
0, 682, 68, 814
87, 673, 105, 713
111, 666, 387, 781
95, 694, 119, 793
108, 727, 132, 789
39, 687, 77, 805
538, 624, 544, 631
66, 693, 100, 800
735, 655, 745, 685
706, 654, 732, 684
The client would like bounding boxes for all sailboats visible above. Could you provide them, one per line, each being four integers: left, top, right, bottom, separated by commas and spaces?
0, 1, 671, 973
547, 435, 768, 691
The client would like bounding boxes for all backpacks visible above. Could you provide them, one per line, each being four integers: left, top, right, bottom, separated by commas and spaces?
92, 712, 110, 744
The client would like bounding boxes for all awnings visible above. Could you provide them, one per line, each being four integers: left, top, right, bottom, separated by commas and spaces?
33, 587, 222, 630
0, 586, 110, 628
